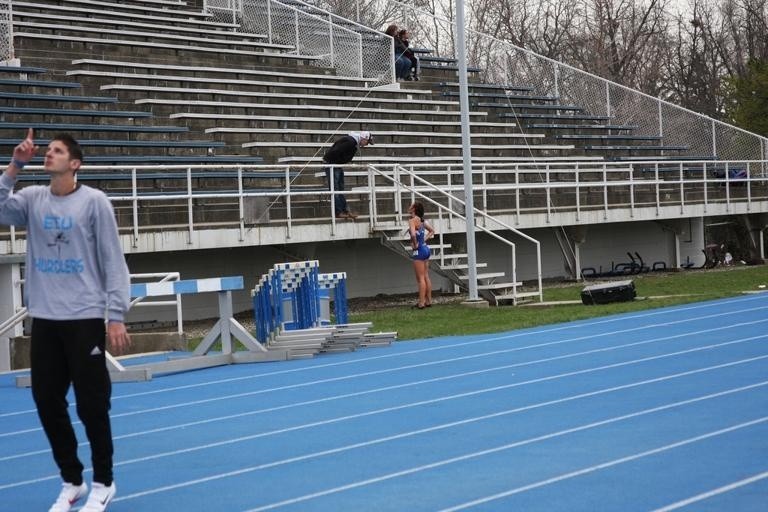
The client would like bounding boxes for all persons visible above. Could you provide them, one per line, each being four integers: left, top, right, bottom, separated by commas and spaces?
323, 130, 373, 218
408, 202, 436, 308
1, 125, 135, 511
399, 27, 420, 82
384, 25, 412, 82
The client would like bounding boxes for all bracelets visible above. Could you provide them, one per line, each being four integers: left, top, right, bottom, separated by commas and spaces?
10, 157, 27, 169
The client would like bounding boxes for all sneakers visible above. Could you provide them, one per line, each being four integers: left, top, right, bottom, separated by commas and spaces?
335, 212, 356, 218
79, 481, 116, 512
411, 303, 433, 309
48, 481, 88, 512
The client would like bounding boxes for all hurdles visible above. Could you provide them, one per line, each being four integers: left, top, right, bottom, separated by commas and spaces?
251, 259, 398, 358
15, 275, 289, 387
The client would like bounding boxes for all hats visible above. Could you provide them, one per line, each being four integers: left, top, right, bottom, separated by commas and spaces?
361, 131, 374, 145
